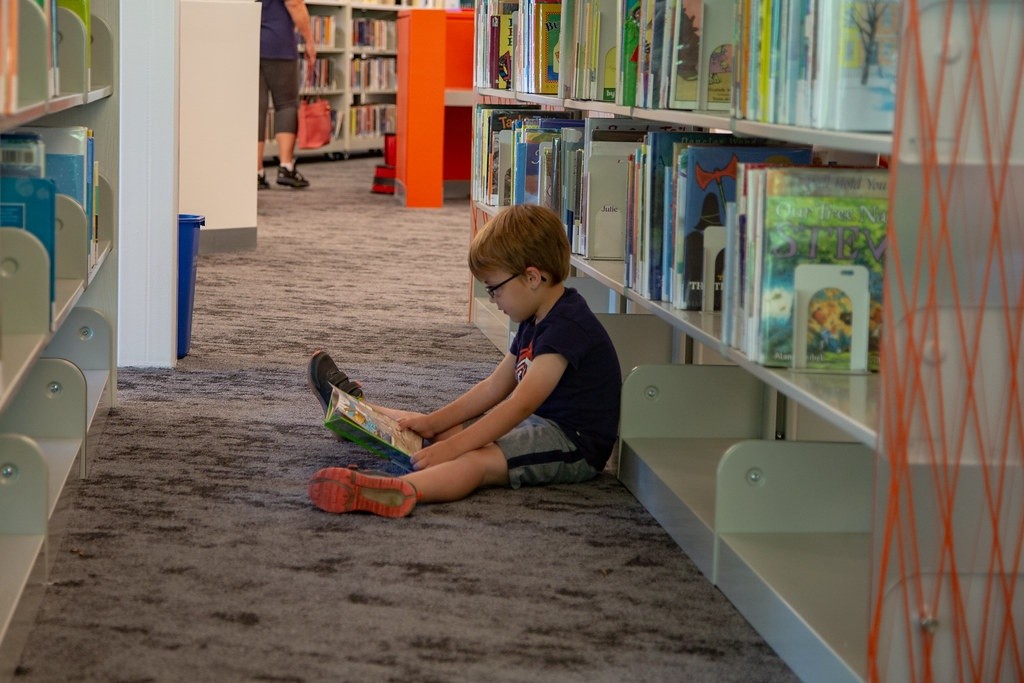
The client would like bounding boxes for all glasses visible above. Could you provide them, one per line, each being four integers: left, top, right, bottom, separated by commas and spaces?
486, 271, 547, 299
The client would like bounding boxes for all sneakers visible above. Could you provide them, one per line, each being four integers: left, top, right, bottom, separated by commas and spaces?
307, 350, 363, 421
258, 172, 270, 190
310, 467, 418, 519
276, 160, 310, 187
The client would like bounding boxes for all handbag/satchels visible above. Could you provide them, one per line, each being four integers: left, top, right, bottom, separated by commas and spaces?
297, 63, 331, 149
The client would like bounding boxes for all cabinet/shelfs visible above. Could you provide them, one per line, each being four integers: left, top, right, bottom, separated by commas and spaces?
1, 0, 1024, 683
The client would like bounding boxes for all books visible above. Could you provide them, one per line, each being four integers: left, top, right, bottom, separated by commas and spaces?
471, 0, 900, 370
264, 0, 475, 143
0, 0, 98, 333
325, 381, 424, 472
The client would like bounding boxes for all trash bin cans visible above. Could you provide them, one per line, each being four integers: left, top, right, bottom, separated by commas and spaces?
178, 214, 206, 360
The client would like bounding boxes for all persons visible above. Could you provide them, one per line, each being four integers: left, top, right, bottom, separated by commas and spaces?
255, 0, 316, 187
307, 204, 622, 517
